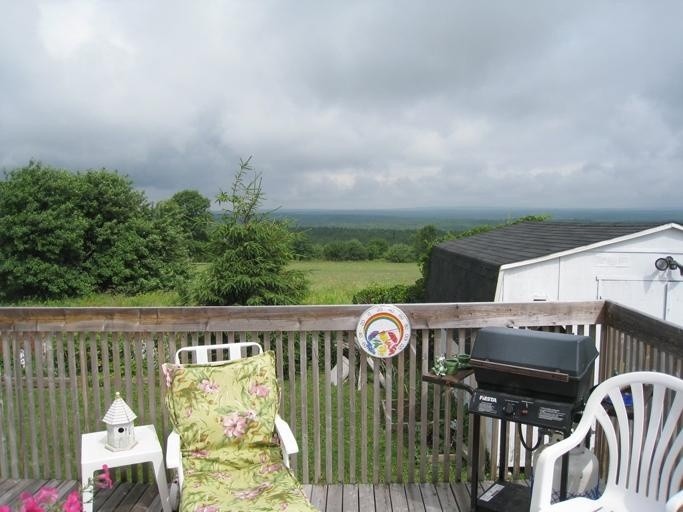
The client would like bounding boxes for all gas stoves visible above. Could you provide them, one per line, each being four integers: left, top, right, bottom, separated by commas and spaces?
468, 327, 601, 438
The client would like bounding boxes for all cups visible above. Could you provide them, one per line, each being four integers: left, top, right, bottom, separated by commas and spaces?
444, 359, 459, 376
459, 355, 470, 365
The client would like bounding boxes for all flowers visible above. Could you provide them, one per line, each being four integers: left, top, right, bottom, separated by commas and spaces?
0, 465, 113, 512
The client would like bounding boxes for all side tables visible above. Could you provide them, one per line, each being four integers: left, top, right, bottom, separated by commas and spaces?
81, 424, 171, 512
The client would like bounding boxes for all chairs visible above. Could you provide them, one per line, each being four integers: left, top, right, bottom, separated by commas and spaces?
530, 371, 683, 512
162, 342, 319, 512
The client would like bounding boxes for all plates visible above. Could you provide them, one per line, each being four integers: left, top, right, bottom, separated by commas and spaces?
606, 391, 635, 407
